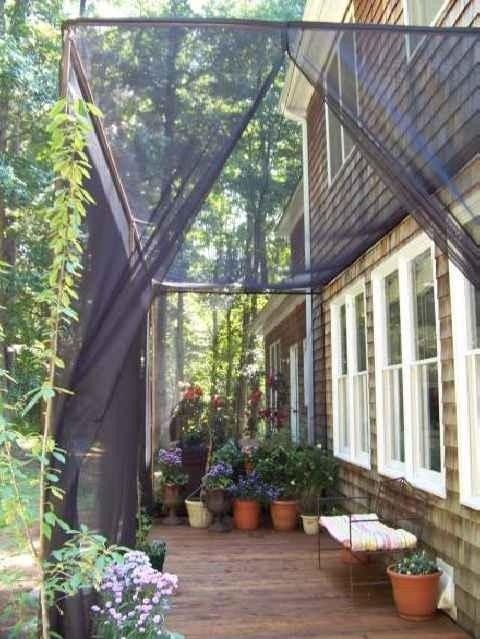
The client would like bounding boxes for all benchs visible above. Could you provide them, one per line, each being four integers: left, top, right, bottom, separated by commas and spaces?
315, 476, 432, 595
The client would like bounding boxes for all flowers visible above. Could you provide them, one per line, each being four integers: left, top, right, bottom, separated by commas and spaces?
95, 547, 187, 639
149, 379, 331, 537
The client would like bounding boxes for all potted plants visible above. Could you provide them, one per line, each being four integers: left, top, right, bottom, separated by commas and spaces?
386, 547, 440, 623
128, 539, 164, 577
